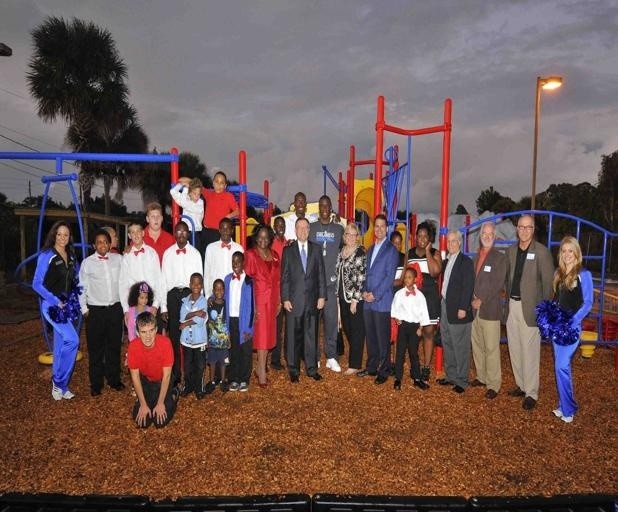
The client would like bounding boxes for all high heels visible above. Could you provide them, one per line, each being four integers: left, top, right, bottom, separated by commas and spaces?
422, 367, 430, 381
258, 378, 267, 389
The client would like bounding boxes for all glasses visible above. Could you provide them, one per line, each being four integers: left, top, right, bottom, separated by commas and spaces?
519, 226, 533, 230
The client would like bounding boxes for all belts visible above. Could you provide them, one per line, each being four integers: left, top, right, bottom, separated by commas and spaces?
510, 295, 521, 301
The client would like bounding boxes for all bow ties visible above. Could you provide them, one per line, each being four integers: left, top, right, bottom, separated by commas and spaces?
176, 248, 186, 254
232, 275, 240, 280
99, 257, 108, 260
221, 242, 231, 250
406, 291, 415, 296
134, 248, 144, 256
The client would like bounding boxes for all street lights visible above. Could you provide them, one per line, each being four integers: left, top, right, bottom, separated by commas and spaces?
531, 74, 564, 218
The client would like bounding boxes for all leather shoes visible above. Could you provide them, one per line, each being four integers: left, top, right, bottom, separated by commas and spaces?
326, 358, 341, 373
357, 370, 376, 376
91, 387, 102, 395
485, 389, 496, 398
308, 373, 322, 380
523, 396, 535, 409
109, 381, 125, 390
414, 379, 429, 389
508, 388, 526, 396
470, 379, 485, 385
343, 368, 359, 375
376, 377, 387, 384
394, 379, 401, 389
436, 378, 448, 384
452, 386, 464, 392
291, 376, 299, 383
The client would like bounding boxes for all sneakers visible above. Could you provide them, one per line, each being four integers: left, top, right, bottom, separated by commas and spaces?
239, 381, 248, 391
63, 391, 75, 399
220, 380, 228, 391
196, 392, 203, 400
561, 415, 573, 423
229, 382, 239, 391
206, 381, 215, 391
52, 380, 62, 401
181, 389, 191, 396
552, 408, 562, 417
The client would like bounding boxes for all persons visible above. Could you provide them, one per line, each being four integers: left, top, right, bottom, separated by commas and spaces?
551, 236, 594, 424
169, 177, 205, 252
435, 227, 477, 394
470, 219, 512, 400
179, 170, 240, 262
77, 194, 446, 399
30, 220, 84, 403
505, 214, 555, 412
125, 311, 180, 429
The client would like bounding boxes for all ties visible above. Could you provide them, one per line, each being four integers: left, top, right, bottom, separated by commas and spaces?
301, 245, 307, 273
